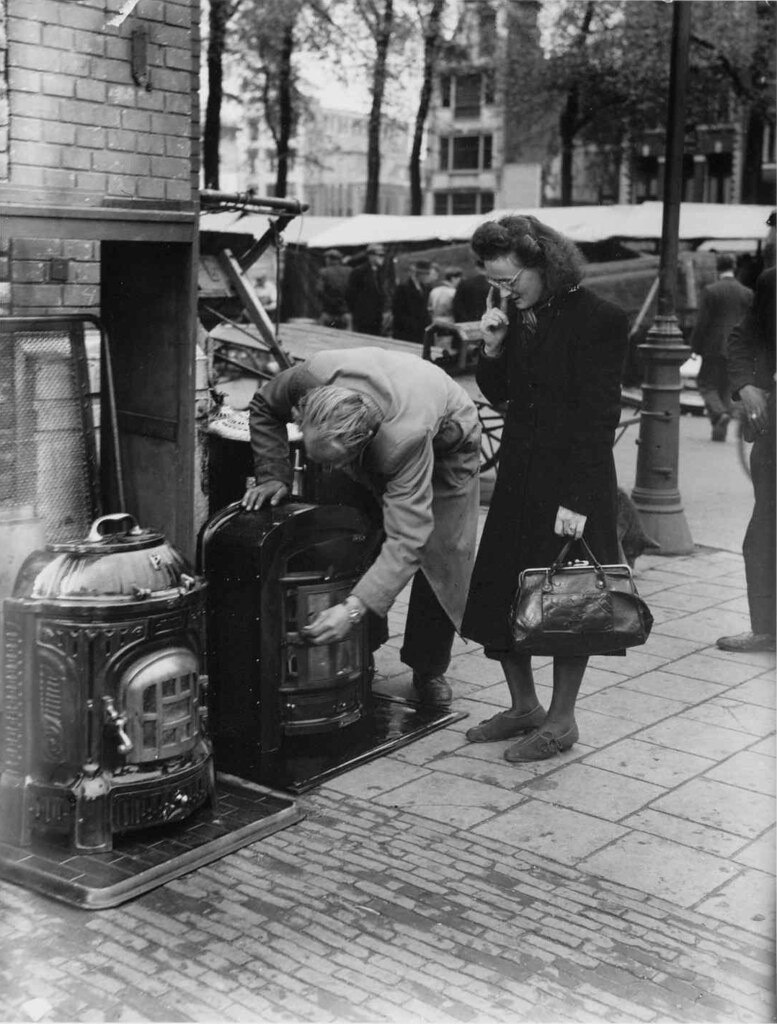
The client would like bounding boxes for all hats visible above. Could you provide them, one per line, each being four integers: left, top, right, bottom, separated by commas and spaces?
324, 250, 342, 258
409, 258, 431, 273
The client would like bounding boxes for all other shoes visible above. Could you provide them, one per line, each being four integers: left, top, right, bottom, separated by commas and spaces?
711, 413, 730, 442
412, 669, 452, 706
466, 704, 548, 742
716, 631, 777, 653
504, 718, 579, 763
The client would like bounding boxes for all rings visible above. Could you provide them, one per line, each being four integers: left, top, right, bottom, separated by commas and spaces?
569, 527, 576, 533
752, 414, 756, 419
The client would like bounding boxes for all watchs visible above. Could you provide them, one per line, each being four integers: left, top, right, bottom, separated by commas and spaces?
342, 600, 363, 625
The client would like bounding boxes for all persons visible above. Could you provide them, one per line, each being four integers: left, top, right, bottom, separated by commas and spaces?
459, 216, 632, 763
716, 209, 777, 651
309, 244, 504, 372
243, 344, 482, 705
689, 252, 752, 442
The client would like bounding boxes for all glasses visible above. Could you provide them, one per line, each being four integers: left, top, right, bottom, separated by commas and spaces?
484, 265, 530, 291
369, 251, 385, 257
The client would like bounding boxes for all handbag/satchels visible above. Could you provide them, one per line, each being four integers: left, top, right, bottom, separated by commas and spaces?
510, 533, 654, 657
